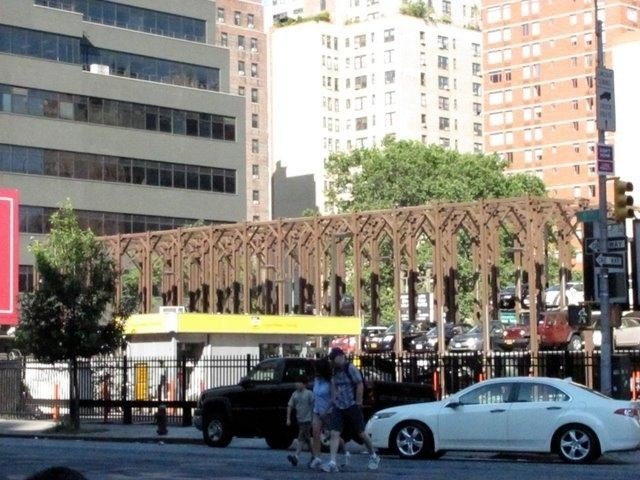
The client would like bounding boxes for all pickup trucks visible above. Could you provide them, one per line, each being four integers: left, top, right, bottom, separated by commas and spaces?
189, 355, 440, 450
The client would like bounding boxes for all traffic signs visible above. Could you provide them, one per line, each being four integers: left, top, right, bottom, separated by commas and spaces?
585, 237, 626, 255
594, 253, 623, 269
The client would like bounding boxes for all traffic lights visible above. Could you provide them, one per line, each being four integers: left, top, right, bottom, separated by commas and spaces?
611, 176, 634, 222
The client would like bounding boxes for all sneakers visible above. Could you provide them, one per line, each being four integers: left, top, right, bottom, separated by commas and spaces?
367, 455, 380, 470
287, 451, 350, 472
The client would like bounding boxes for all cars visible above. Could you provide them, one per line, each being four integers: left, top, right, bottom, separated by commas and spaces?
361, 375, 638, 464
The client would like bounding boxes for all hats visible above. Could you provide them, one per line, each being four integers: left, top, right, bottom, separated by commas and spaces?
329, 347, 344, 360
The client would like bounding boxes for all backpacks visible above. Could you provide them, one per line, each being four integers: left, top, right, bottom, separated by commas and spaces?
343, 362, 368, 401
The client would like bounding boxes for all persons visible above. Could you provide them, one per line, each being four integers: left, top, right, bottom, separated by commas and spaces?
310, 358, 352, 469
286, 373, 314, 466
318, 345, 381, 472
